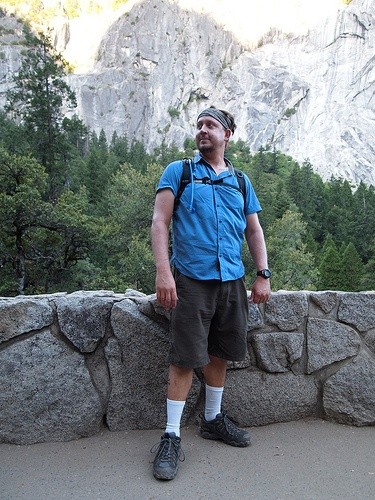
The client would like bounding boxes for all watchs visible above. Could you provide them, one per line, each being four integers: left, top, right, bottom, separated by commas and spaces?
257, 269, 272, 280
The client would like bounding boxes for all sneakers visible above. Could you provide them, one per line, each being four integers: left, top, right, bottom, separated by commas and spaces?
150, 431, 185, 481
198, 411, 251, 448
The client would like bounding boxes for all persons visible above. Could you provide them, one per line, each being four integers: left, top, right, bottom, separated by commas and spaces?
149, 106, 272, 481
